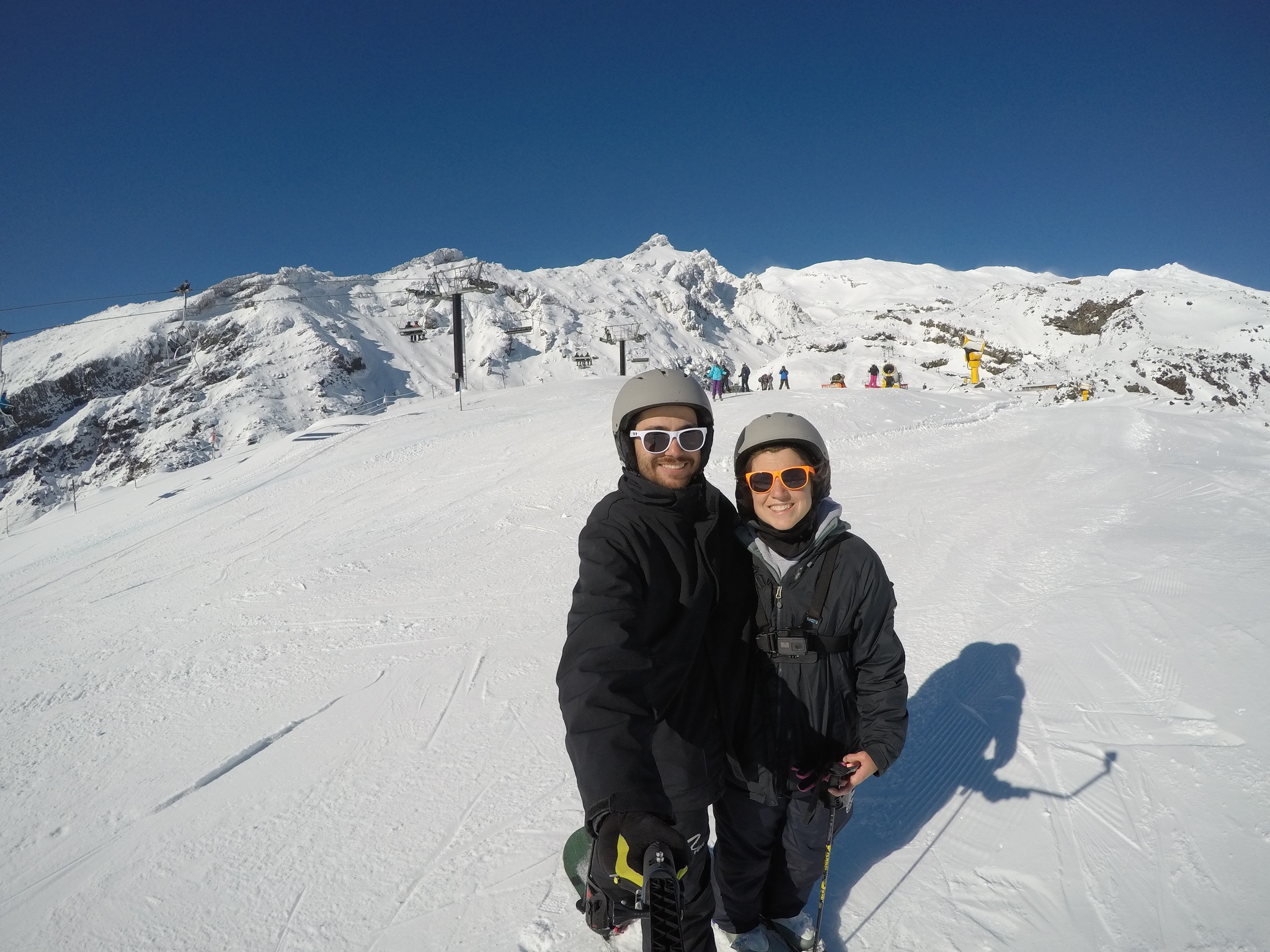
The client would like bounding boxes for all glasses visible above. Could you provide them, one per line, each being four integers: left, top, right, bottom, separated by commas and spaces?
713, 362, 717, 364
745, 466, 815, 494
629, 427, 707, 454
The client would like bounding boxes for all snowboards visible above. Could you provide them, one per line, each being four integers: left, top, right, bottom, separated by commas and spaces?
821, 383, 842, 388
563, 826, 643, 952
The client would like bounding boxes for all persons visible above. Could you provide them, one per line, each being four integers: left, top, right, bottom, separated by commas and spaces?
730, 412, 908, 952
554, 367, 755, 952
704, 360, 880, 402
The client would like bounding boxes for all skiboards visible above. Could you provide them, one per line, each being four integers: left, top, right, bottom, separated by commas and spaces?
407, 336, 435, 344
574, 364, 597, 367
712, 395, 723, 402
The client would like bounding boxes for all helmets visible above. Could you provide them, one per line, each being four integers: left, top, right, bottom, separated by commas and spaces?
758, 377, 763, 382
742, 362, 746, 365
734, 412, 831, 516
612, 369, 714, 475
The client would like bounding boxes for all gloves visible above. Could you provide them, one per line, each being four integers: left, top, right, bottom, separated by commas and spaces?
788, 728, 840, 792
589, 810, 691, 911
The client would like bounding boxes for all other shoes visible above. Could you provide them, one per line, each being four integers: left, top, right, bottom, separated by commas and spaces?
759, 909, 816, 950
719, 396, 722, 398
726, 926, 772, 952
713, 396, 715, 398
577, 364, 593, 366
411, 337, 426, 342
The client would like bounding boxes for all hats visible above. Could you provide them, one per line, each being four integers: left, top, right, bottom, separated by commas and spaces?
713, 359, 717, 363
